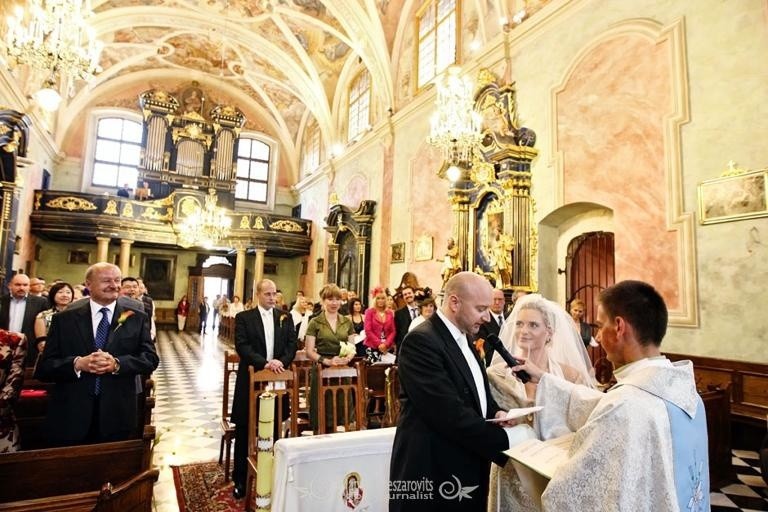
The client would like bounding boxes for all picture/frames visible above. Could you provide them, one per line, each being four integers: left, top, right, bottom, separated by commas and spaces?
697, 169, 768, 225
415, 237, 433, 261
316, 258, 324, 274
113, 253, 135, 267
264, 263, 278, 273
391, 243, 406, 262
300, 260, 309, 275
67, 249, 91, 265
138, 251, 178, 302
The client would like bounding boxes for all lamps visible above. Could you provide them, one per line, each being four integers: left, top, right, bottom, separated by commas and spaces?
173, 0, 235, 253
0, 0, 105, 115
428, 62, 485, 159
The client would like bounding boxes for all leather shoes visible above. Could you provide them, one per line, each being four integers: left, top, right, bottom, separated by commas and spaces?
233, 481, 245, 496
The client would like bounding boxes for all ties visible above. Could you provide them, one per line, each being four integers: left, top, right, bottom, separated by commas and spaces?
499, 316, 502, 326
94, 308, 110, 395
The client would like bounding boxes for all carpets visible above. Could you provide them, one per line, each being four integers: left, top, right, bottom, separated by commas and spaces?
169, 458, 255, 511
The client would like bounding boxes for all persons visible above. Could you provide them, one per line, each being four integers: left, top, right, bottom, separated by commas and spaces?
117, 184, 129, 209
139, 182, 150, 200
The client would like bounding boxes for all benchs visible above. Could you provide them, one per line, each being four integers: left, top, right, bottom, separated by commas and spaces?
0, 365, 161, 511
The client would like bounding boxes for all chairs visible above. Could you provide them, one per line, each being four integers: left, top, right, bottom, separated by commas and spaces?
216, 350, 402, 511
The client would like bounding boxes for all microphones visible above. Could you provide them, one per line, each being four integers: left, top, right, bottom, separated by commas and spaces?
486, 330, 533, 387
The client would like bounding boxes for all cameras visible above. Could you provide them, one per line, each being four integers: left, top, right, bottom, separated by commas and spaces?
362, 346, 377, 366
